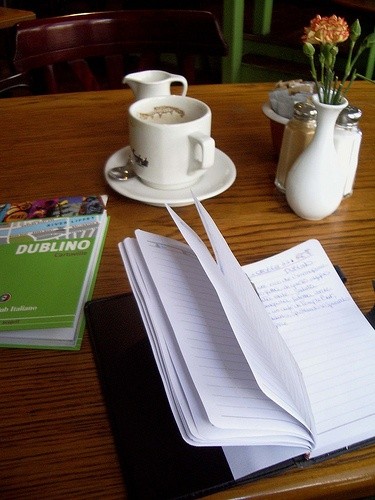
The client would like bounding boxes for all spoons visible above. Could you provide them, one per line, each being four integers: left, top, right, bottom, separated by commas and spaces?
109, 160, 137, 182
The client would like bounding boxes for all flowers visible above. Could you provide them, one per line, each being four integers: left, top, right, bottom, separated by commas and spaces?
300, 13, 375, 104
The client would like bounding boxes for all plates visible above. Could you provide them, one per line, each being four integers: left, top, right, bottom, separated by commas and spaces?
102, 143, 238, 210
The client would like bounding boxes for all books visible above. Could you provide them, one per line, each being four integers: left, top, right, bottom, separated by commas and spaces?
0, 192, 112, 351
84, 188, 375, 499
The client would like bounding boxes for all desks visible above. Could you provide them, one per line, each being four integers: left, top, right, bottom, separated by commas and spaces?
0, 77, 374, 500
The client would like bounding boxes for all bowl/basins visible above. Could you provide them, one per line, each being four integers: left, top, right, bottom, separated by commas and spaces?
261, 98, 288, 157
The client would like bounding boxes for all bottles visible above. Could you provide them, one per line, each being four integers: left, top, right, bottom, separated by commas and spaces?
273, 101, 317, 195
332, 104, 364, 199
284, 90, 349, 221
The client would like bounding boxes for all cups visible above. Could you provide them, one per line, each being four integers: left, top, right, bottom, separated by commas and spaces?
122, 70, 191, 103
128, 95, 216, 191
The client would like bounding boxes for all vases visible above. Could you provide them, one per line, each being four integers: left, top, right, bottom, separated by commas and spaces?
286, 90, 349, 222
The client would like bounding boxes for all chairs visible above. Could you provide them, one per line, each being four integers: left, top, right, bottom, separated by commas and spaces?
0, 7, 232, 98
